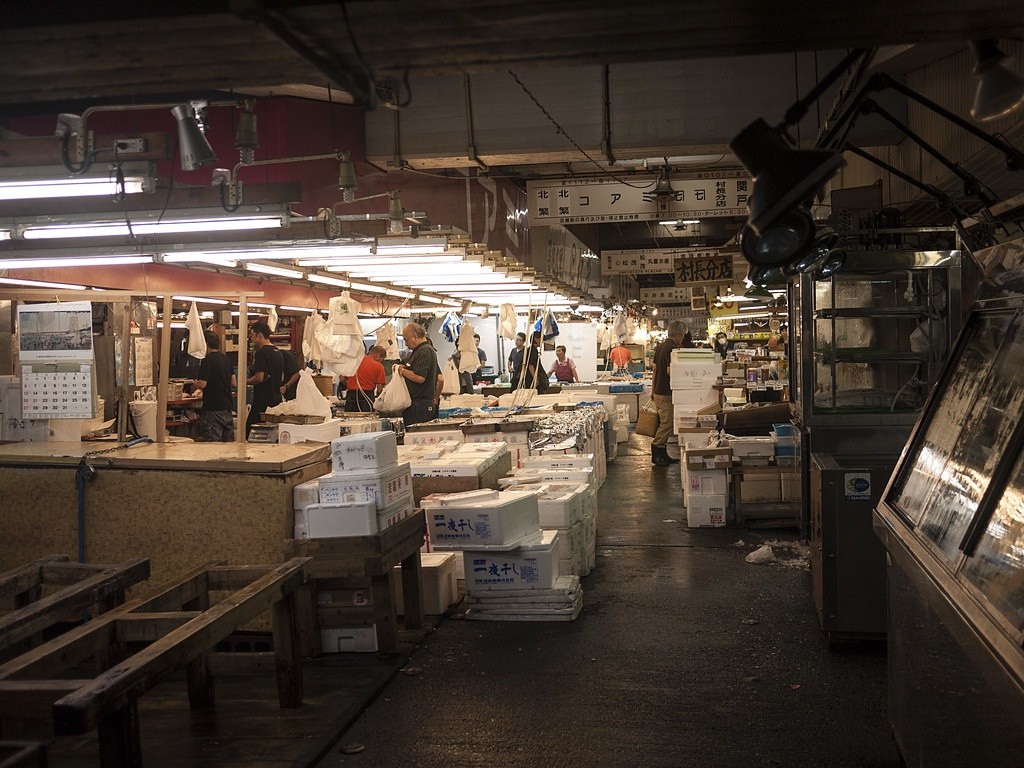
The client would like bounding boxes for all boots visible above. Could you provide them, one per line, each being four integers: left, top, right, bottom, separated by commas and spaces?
652, 445, 670, 466
663, 447, 679, 463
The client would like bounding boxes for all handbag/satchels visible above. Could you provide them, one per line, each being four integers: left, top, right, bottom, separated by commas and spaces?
374, 364, 412, 413
636, 399, 659, 438
441, 361, 460, 395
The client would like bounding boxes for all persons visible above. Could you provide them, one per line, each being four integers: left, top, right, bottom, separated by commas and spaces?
506, 332, 549, 393
448, 334, 487, 393
650, 320, 688, 466
353, 594, 367, 604
343, 346, 387, 412
246, 321, 284, 439
717, 332, 733, 358
546, 346, 579, 383
769, 325, 787, 350
609, 342, 636, 375
192, 330, 237, 441
278, 348, 301, 402
393, 324, 444, 424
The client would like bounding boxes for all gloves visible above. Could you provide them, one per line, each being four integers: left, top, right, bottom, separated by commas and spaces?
183, 383, 196, 394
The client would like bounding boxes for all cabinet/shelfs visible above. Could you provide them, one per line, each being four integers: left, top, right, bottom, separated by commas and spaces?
786, 221, 964, 427
247, 316, 302, 354
165, 396, 204, 435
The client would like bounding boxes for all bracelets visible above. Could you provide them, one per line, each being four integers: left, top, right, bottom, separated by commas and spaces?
399, 369, 404, 376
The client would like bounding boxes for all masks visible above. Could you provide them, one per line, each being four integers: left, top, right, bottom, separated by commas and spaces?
718, 339, 725, 344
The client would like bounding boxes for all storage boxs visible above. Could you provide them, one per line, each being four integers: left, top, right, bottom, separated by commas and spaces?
670, 347, 801, 528
279, 382, 644, 654
157, 383, 183, 401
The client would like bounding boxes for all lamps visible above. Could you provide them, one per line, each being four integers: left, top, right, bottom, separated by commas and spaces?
171, 93, 219, 172
738, 43, 1024, 302
3, 161, 607, 313
